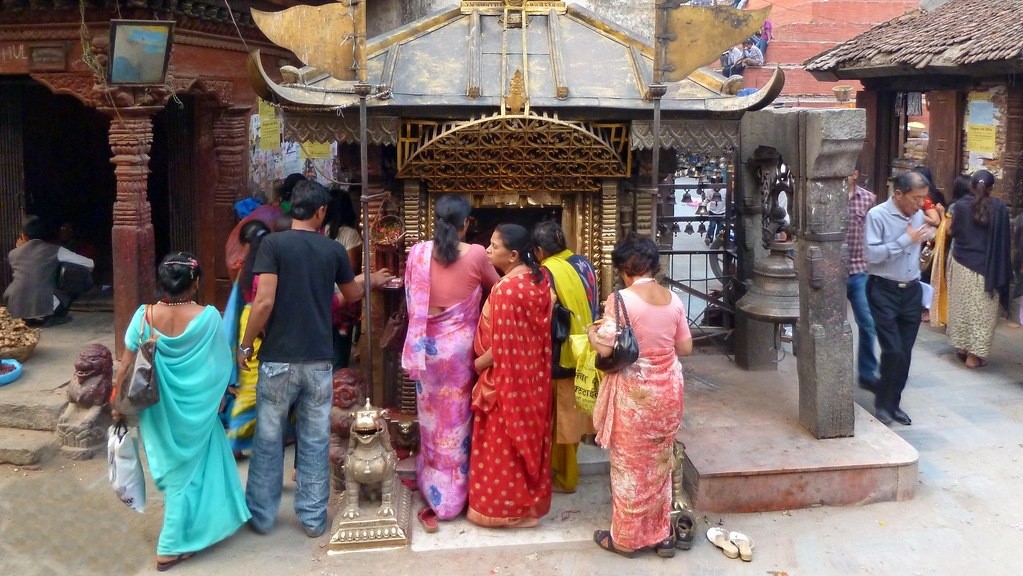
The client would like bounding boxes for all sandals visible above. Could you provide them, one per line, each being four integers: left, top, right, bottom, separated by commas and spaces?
594, 529, 638, 559
157, 550, 195, 572
418, 505, 439, 533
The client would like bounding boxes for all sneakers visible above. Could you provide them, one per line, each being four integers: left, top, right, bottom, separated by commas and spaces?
657, 522, 674, 558
675, 513, 696, 551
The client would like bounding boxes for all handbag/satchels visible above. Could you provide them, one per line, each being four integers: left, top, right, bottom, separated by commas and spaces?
595, 326, 639, 374
127, 336, 159, 402
226, 202, 278, 285
568, 334, 605, 417
107, 415, 147, 514
552, 303, 574, 344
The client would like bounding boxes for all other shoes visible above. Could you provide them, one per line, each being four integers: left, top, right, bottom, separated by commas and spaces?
45, 316, 72, 327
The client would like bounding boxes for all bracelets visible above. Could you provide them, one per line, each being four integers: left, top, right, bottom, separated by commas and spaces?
588, 331, 595, 343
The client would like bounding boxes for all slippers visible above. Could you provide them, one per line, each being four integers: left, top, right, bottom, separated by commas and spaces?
729, 530, 754, 562
706, 527, 739, 559
967, 357, 988, 369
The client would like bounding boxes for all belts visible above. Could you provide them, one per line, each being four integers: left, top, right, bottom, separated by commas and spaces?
873, 275, 918, 288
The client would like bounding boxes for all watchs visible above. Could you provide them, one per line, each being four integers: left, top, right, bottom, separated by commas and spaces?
240, 343, 254, 355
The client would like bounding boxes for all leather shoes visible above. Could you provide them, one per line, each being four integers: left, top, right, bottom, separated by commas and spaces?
859, 380, 879, 393
877, 404, 892, 424
892, 408, 912, 425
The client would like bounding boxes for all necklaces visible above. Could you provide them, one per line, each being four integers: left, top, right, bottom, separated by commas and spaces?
156, 300, 197, 306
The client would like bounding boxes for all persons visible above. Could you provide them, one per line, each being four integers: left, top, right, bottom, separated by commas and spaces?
722, 20, 772, 78
2, 217, 94, 328
701, 165, 1023, 425
110, 173, 692, 570
